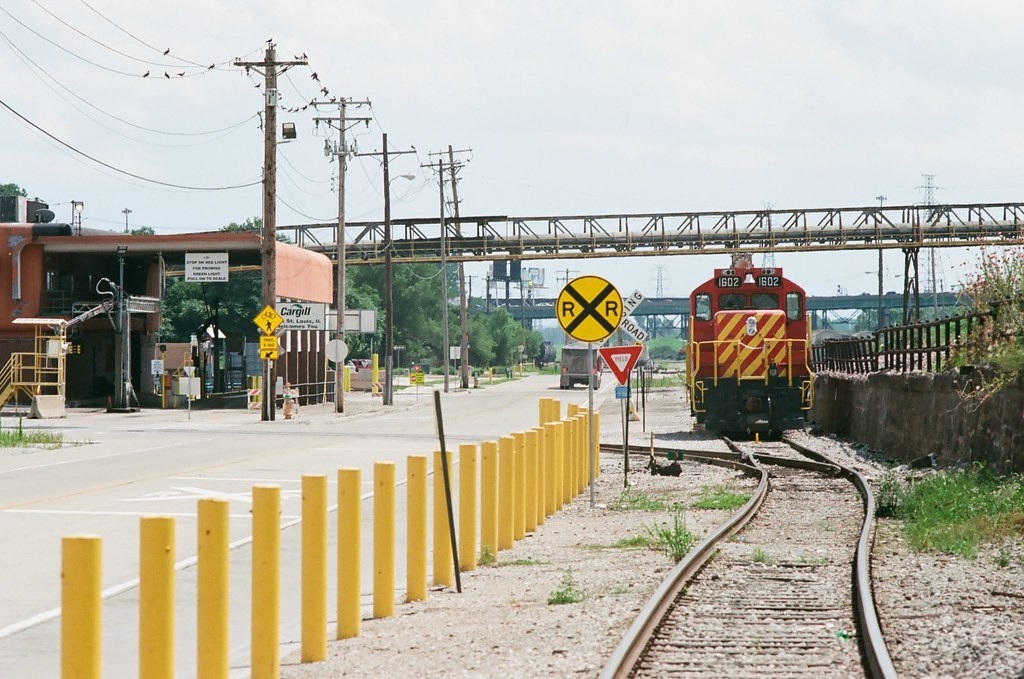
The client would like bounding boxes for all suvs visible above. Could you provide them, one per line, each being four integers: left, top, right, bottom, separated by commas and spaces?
559, 344, 604, 391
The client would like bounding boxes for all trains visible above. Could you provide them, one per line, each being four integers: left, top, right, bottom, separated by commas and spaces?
681, 265, 814, 441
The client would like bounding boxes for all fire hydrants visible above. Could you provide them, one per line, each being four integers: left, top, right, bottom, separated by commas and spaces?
282, 398, 296, 420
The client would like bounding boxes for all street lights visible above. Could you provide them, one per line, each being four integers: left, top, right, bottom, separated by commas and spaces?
866, 270, 887, 330
262, 122, 299, 422
114, 244, 128, 408
383, 173, 419, 407
74, 201, 84, 238
896, 273, 911, 328
